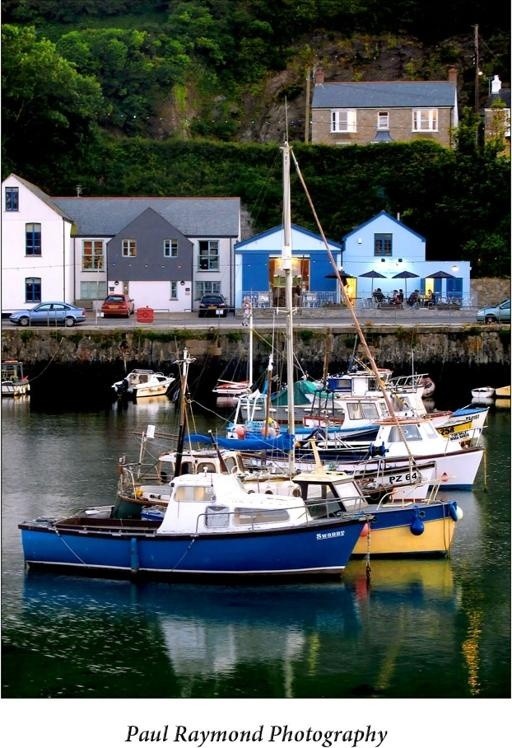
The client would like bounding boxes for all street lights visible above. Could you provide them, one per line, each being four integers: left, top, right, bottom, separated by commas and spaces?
473, 65, 486, 152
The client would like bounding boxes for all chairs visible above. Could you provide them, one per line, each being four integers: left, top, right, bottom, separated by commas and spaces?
358, 291, 460, 314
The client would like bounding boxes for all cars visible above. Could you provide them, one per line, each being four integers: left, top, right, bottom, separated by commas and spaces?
9, 301, 86, 327
476, 297, 510, 324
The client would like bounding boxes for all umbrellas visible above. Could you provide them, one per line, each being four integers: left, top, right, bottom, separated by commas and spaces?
325, 272, 356, 281
358, 270, 386, 297
390, 270, 420, 304
424, 270, 456, 303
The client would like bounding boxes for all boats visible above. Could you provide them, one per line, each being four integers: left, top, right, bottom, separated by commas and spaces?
17, 570, 373, 696
496, 384, 510, 396
494, 398, 510, 408
0, 357, 34, 396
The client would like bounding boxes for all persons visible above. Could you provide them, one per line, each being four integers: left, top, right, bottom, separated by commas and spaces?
373, 288, 433, 306
240, 296, 252, 326
